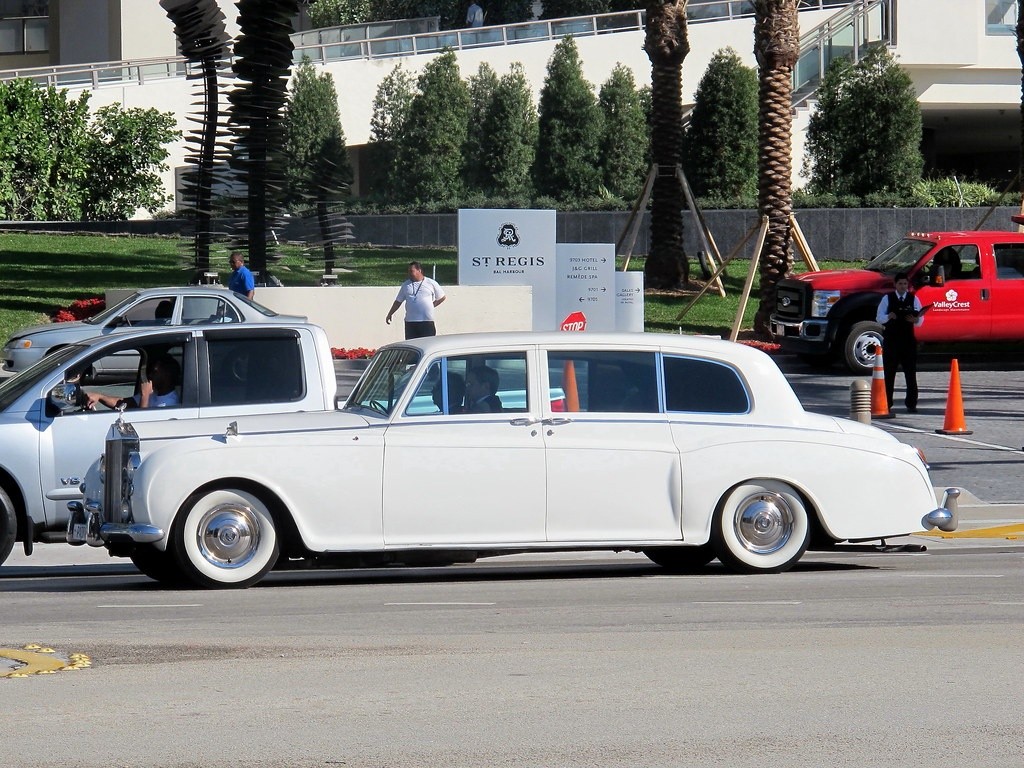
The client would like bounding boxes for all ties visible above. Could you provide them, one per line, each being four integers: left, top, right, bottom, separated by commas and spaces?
900, 296, 903, 301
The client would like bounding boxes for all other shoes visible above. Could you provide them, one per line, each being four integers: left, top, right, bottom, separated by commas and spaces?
906, 406, 918, 413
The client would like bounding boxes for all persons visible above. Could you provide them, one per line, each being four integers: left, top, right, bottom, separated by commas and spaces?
431, 371, 464, 412
228, 252, 255, 300
80, 351, 182, 410
386, 261, 446, 381
464, 365, 502, 413
155, 300, 173, 324
465, 0, 484, 29
875, 273, 925, 414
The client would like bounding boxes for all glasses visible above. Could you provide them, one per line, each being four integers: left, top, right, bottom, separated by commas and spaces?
147, 368, 165, 378
464, 381, 472, 388
228, 259, 243, 264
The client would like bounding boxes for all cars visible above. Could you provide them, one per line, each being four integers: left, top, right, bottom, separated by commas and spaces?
64, 330, 961, 591
1, 286, 310, 387
768, 228, 1024, 375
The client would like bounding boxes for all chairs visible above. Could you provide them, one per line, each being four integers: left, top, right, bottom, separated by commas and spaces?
970, 252, 982, 280
932, 245, 963, 276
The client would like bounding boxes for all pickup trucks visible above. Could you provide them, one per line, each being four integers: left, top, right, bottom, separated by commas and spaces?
0, 324, 567, 568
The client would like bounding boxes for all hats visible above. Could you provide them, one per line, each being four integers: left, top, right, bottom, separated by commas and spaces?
155, 354, 181, 374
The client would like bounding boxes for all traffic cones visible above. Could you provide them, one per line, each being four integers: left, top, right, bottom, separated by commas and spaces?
564, 359, 579, 412
869, 344, 895, 421
936, 359, 973, 435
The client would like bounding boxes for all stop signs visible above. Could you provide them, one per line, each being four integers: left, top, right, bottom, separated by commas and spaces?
560, 310, 587, 331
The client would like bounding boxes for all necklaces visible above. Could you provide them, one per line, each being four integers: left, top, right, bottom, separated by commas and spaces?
412, 278, 424, 296
